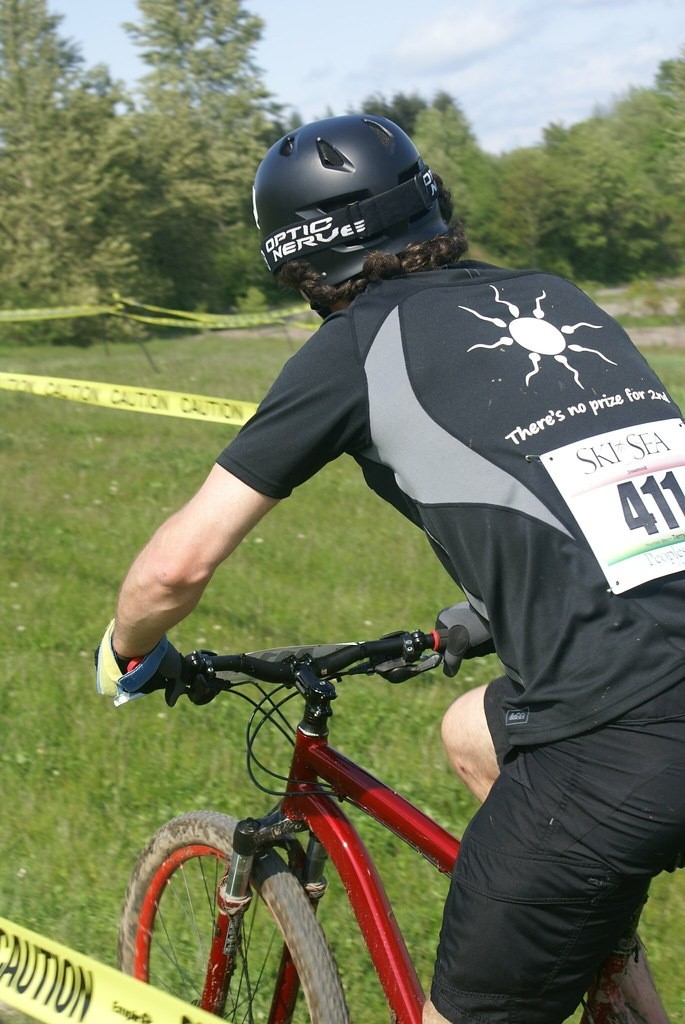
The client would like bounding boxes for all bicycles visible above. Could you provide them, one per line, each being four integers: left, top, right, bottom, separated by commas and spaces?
94, 618, 679, 1024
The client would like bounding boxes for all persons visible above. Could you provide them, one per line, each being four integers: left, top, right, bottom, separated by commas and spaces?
97, 114, 685, 1024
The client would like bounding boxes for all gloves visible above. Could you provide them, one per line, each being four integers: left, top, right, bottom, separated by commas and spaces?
433, 600, 496, 677
93, 617, 195, 708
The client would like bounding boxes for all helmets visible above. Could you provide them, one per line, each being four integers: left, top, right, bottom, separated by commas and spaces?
251, 113, 450, 287
